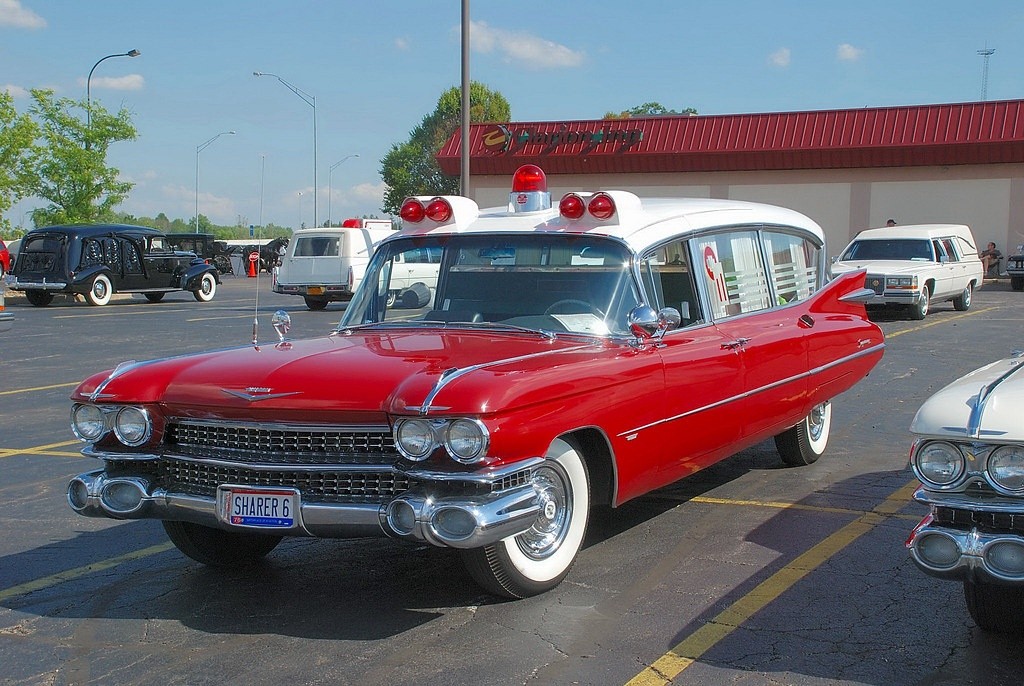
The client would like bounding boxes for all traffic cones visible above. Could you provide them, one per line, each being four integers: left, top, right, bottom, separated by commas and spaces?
248, 262, 257, 277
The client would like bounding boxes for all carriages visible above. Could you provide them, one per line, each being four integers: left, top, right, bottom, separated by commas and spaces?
212, 236, 290, 274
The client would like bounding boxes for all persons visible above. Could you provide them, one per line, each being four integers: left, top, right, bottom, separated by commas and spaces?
887, 219, 897, 227
979, 242, 1002, 277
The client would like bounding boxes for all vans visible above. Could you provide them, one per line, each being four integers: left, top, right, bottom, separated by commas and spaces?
270, 217, 446, 309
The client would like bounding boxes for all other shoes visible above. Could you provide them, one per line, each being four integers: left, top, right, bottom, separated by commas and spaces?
983, 271, 990, 277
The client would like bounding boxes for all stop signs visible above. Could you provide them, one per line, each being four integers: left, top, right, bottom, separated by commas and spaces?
248, 252, 259, 261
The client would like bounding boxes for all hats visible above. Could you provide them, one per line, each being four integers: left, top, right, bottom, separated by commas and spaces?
886, 219, 897, 225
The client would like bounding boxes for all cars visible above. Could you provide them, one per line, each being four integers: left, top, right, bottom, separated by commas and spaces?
162, 233, 214, 268
1007, 242, 1024, 290
0, 240, 11, 278
4, 224, 223, 306
903, 343, 1024, 637
65, 162, 886, 600
829, 224, 984, 318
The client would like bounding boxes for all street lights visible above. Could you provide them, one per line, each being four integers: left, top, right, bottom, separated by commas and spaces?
253, 71, 317, 229
88, 49, 140, 147
329, 154, 360, 227
194, 131, 237, 233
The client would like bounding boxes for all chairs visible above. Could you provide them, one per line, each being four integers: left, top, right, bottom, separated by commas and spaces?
424, 310, 484, 322
987, 257, 1004, 276
